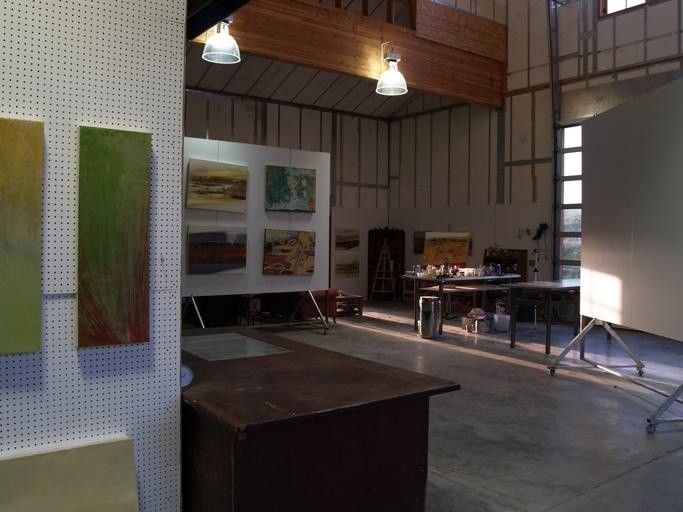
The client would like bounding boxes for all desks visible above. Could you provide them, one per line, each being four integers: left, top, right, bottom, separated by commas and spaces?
401, 273, 612, 353
180, 325, 460, 507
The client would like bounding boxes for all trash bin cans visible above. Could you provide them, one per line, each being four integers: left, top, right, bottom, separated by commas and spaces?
419, 296, 439, 338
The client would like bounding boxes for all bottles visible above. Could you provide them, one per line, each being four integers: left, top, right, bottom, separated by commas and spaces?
496, 264, 502, 276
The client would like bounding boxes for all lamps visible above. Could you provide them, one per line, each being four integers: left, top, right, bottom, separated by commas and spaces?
375, 41, 408, 96
202, 14, 241, 66
